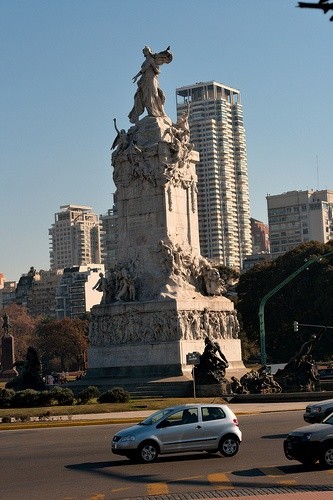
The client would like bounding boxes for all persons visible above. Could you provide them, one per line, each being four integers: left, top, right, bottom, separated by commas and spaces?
87, 308, 240, 346
164, 99, 194, 162
2, 312, 11, 335
60, 375, 82, 384
93, 267, 138, 304
151, 240, 227, 298
128, 45, 173, 124
111, 119, 128, 166
191, 322, 328, 394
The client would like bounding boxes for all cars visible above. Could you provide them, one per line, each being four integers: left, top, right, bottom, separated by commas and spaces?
303, 397, 333, 424
110, 403, 242, 463
283, 411, 333, 469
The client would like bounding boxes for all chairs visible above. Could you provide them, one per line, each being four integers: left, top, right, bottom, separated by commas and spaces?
187, 412, 197, 423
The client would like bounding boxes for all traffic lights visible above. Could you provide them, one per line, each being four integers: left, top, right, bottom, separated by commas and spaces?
293, 321, 299, 332
